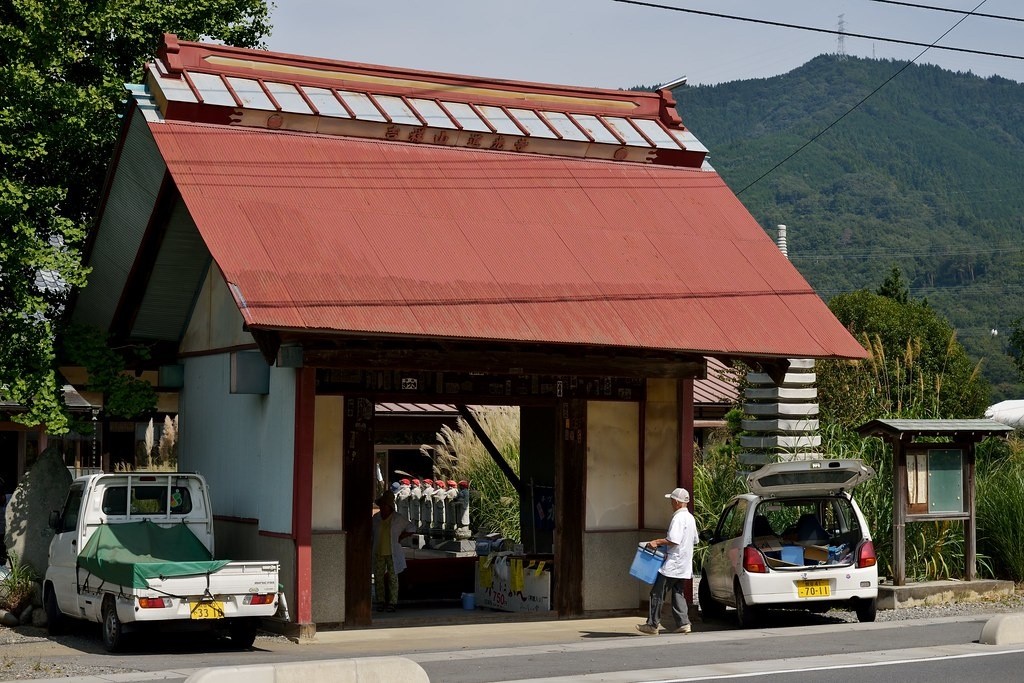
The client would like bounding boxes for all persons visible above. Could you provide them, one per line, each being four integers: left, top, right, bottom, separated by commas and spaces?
636, 488, 700, 636
395, 478, 470, 531
371, 492, 417, 614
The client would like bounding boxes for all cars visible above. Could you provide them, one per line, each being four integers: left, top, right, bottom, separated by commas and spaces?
698, 459, 879, 628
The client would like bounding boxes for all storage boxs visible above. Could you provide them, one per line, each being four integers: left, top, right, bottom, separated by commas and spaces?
628, 541, 667, 585
463, 593, 475, 610
804, 544, 829, 562
765, 547, 804, 567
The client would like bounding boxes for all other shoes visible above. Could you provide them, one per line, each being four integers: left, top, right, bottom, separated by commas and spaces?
386, 604, 397, 613
376, 602, 385, 612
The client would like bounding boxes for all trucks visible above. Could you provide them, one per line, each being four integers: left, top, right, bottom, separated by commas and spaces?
42, 471, 281, 656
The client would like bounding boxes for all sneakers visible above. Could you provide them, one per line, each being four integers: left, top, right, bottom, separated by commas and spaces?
664, 624, 691, 633
635, 624, 658, 635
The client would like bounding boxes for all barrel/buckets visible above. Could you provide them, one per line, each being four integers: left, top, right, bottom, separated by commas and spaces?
461, 593, 476, 609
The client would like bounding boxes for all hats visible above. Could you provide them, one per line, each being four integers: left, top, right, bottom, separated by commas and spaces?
665, 488, 689, 503
375, 478, 469, 510
